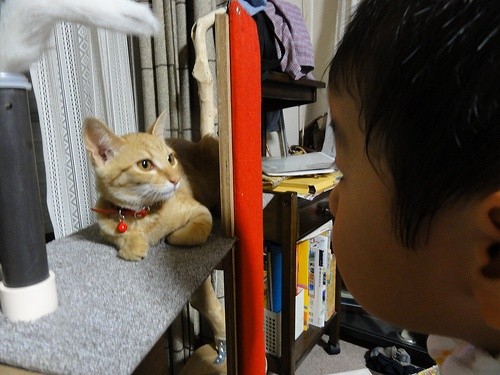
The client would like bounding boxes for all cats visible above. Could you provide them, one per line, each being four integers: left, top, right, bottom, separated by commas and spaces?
81, 109, 221, 261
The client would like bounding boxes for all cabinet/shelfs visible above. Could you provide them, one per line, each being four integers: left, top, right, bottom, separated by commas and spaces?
235, 72, 348, 375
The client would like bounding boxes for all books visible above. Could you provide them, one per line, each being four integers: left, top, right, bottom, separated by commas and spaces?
261, 222, 339, 342
261, 151, 335, 178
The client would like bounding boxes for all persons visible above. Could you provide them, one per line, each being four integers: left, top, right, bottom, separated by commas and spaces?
325, 0, 500, 374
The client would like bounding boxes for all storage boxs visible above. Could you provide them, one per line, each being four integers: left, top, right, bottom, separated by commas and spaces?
255, 286, 306, 356
